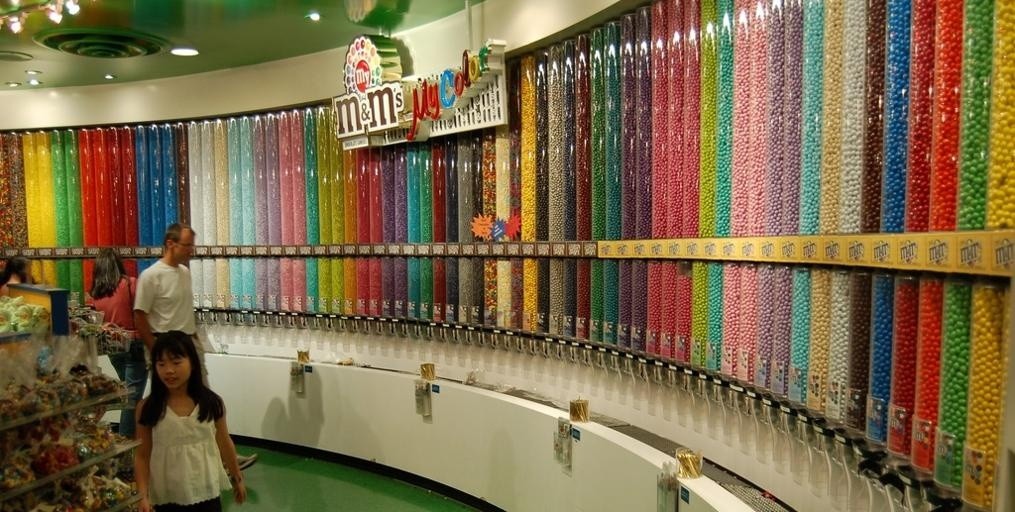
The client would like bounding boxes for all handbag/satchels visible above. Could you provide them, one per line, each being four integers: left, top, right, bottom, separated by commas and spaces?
129, 342, 147, 367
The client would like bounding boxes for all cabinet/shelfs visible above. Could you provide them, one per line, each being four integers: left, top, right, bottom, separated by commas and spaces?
1, 283, 142, 512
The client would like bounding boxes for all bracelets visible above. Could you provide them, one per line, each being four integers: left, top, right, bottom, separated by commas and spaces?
227, 472, 246, 484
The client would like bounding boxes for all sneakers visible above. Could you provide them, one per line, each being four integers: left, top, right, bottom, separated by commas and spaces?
221, 451, 260, 478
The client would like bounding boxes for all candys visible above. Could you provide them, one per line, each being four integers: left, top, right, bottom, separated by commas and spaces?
0, 0, 1015, 512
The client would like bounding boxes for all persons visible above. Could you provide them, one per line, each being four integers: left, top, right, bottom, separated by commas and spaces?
134, 328, 246, 511
86, 247, 148, 482
0, 256, 33, 298
132, 222, 209, 388
